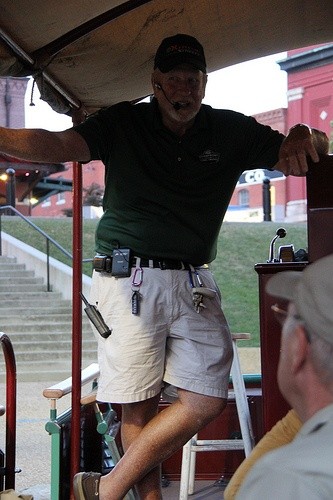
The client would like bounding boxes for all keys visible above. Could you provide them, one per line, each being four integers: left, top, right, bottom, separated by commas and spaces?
193, 295, 207, 313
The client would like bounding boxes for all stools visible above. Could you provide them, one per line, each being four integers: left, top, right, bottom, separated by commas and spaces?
178, 333, 254, 500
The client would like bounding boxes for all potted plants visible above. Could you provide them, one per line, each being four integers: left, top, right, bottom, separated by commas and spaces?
82, 183, 104, 219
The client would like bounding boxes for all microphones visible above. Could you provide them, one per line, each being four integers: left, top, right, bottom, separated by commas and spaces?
155, 83, 180, 110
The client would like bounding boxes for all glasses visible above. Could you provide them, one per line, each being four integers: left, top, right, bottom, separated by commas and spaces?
269, 302, 312, 344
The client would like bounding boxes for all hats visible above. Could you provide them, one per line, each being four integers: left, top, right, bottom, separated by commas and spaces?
154, 33, 207, 73
265, 254, 333, 346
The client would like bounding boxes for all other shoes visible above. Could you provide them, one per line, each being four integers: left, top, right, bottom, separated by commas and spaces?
74, 471, 102, 500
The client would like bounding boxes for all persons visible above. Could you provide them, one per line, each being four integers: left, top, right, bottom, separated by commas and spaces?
234, 256, 333, 500
0, 34, 330, 500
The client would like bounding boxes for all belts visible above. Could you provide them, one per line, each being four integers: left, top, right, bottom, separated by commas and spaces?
130, 259, 208, 271
